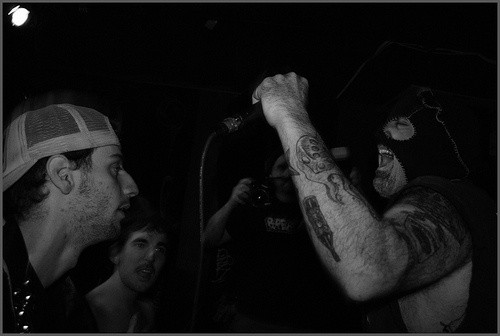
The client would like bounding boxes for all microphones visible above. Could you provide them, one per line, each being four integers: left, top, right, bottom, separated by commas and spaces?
221, 105, 263, 133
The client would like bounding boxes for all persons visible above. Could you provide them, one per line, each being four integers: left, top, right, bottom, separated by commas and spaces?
4, 89, 141, 334
253, 70, 496, 333
84, 207, 170, 334
199, 150, 326, 333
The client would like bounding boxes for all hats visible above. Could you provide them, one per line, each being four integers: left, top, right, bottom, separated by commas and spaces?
1, 104, 119, 192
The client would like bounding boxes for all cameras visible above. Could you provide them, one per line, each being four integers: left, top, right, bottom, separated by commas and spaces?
241, 177, 277, 208
